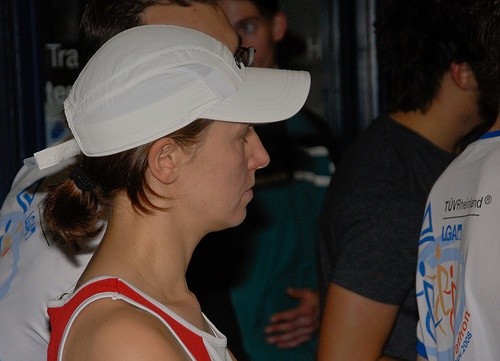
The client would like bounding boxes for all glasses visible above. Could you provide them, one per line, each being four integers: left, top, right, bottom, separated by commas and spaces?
233, 45, 257, 69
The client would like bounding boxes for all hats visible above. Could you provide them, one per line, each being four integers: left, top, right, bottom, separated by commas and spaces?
34, 23, 312, 170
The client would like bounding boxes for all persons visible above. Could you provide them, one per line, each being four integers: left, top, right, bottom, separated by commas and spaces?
43, 22, 312, 361
415, 0, 500, 361
0, 0, 257, 359
316, 0, 486, 360
184, 0, 344, 361
